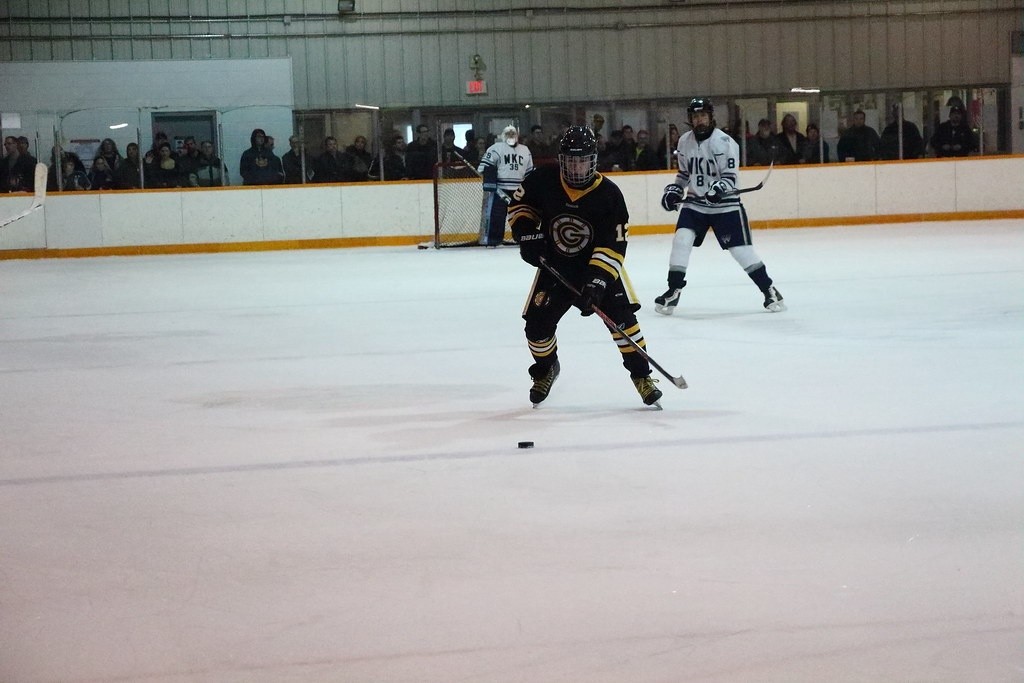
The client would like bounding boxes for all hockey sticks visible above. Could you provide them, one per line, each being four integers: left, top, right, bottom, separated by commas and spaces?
454, 150, 512, 205
539, 256, 688, 391
0, 162, 48, 230
674, 160, 774, 203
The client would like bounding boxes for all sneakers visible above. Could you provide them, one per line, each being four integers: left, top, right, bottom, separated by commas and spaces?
528, 346, 560, 410
623, 361, 663, 411
762, 277, 783, 312
655, 278, 687, 315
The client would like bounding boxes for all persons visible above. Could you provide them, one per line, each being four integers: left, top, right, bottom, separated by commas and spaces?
722, 114, 829, 167
519, 118, 587, 165
655, 98, 786, 315
47, 133, 230, 192
478, 128, 534, 248
837, 104, 923, 162
240, 125, 497, 185
0, 136, 37, 193
593, 114, 679, 171
931, 106, 973, 156
507, 127, 662, 409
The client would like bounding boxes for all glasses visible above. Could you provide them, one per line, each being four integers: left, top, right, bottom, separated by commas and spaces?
4, 141, 15, 146
533, 131, 544, 135
420, 129, 431, 134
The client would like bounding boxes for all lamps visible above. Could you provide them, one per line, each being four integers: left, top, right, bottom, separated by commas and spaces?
338, 0, 355, 16
469, 54, 482, 67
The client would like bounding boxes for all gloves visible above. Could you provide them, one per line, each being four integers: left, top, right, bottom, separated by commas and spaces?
518, 227, 551, 268
576, 278, 607, 316
662, 183, 685, 212
703, 181, 727, 206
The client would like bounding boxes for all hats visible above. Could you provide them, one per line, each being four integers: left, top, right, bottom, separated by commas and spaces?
950, 104, 965, 115
610, 130, 623, 137
289, 135, 299, 144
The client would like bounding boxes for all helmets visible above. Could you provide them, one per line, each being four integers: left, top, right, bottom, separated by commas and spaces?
557, 125, 600, 189
502, 124, 518, 146
687, 97, 717, 141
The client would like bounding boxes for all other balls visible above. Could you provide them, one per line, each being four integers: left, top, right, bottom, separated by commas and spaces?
518, 441, 534, 448
